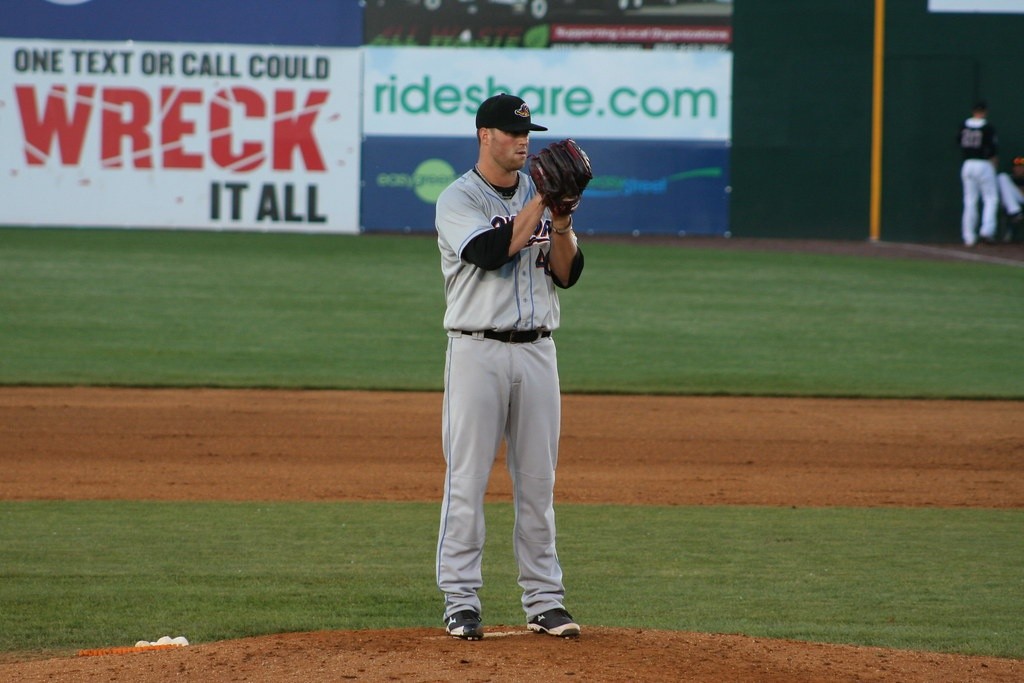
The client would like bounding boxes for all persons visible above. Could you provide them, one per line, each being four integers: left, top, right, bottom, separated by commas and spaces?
997, 157, 1024, 243
435, 92, 594, 638
957, 100, 999, 248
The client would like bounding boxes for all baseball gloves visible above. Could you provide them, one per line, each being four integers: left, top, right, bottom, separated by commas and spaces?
525, 137, 595, 217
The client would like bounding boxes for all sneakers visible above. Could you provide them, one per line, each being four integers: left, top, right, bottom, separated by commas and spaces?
527, 607, 581, 636
442, 611, 485, 640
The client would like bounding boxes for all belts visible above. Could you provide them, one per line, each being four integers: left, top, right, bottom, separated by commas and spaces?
456, 328, 553, 342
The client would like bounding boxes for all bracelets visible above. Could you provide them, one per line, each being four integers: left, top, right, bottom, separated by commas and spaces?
550, 214, 573, 233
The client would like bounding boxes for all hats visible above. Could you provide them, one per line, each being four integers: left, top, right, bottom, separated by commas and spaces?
476, 93, 548, 134
